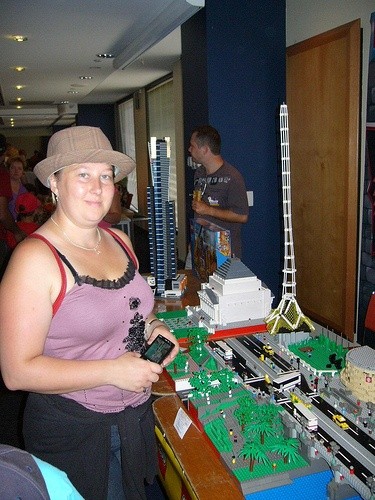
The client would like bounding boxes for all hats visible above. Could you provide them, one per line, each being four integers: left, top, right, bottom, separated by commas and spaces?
15, 192, 41, 213
33, 126, 136, 189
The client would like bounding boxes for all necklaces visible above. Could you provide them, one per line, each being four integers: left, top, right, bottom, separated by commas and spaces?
50, 216, 101, 255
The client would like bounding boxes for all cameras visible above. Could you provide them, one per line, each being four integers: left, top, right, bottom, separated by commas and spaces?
139, 334, 175, 365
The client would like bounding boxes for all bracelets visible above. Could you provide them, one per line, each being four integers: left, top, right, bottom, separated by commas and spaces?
143, 318, 165, 342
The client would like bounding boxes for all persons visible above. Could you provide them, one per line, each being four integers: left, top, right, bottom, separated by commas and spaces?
189, 126, 249, 284
0, 125, 180, 500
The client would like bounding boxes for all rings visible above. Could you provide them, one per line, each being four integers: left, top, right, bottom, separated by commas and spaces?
143, 387, 149, 394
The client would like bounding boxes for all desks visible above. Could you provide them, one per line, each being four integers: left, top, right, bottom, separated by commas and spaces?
112, 207, 135, 252
